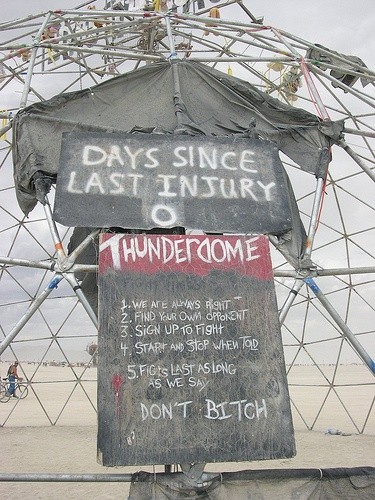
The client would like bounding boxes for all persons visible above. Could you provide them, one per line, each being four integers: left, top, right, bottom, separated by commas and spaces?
6, 360, 20, 399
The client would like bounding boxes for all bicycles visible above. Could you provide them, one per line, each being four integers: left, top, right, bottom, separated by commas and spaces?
0, 382, 11, 403
0, 376, 29, 399
